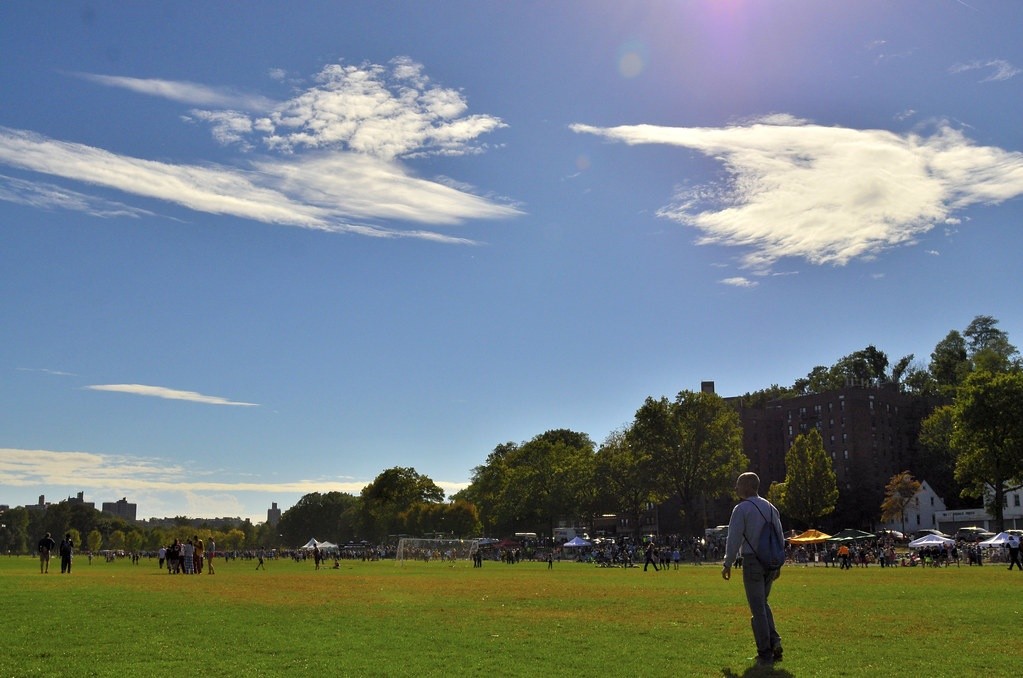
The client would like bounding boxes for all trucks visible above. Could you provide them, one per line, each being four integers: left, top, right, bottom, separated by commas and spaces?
552, 527, 590, 542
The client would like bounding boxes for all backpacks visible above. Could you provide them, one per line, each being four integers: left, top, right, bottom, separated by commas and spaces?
743, 500, 785, 569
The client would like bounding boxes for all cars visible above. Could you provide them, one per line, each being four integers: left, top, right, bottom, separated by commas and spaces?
537, 537, 555, 545
957, 527, 996, 540
592, 534, 656, 545
914, 529, 952, 540
875, 530, 907, 541
1005, 530, 1023, 537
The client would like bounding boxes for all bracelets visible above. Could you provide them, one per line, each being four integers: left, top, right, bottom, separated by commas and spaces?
723, 563, 729, 569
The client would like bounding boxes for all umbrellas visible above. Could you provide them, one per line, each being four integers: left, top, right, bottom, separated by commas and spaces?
789, 528, 877, 550
908, 533, 955, 548
563, 535, 592, 547
978, 532, 1021, 548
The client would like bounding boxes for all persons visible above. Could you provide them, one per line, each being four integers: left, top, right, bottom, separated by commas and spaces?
721, 471, 786, 664
403, 537, 720, 573
255, 543, 403, 571
1005, 535, 1023, 571
38, 531, 74, 575
81, 549, 256, 563
785, 542, 1010, 569
158, 535, 216, 575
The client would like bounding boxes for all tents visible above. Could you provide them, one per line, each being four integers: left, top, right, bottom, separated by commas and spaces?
301, 537, 339, 552
